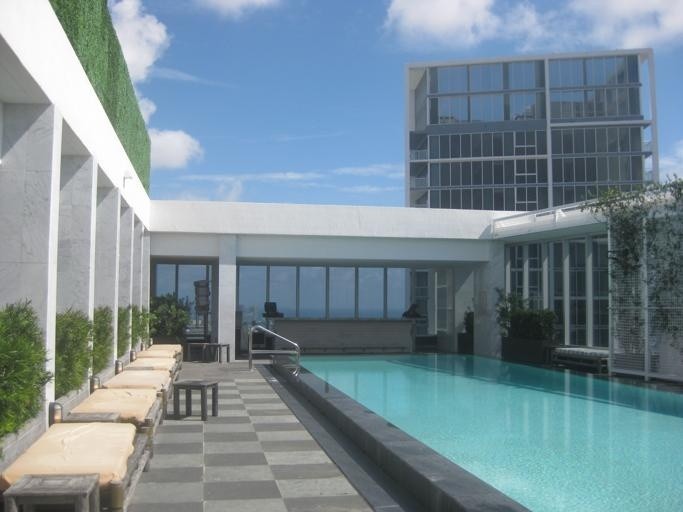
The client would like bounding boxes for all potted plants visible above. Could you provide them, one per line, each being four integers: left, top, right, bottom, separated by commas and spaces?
493, 286, 554, 363
616, 297, 662, 373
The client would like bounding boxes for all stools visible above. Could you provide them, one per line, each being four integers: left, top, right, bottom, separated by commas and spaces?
173, 378, 218, 421
186, 342, 231, 363
0, 343, 183, 512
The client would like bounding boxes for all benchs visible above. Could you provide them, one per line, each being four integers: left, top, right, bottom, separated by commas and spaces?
555, 344, 609, 374
